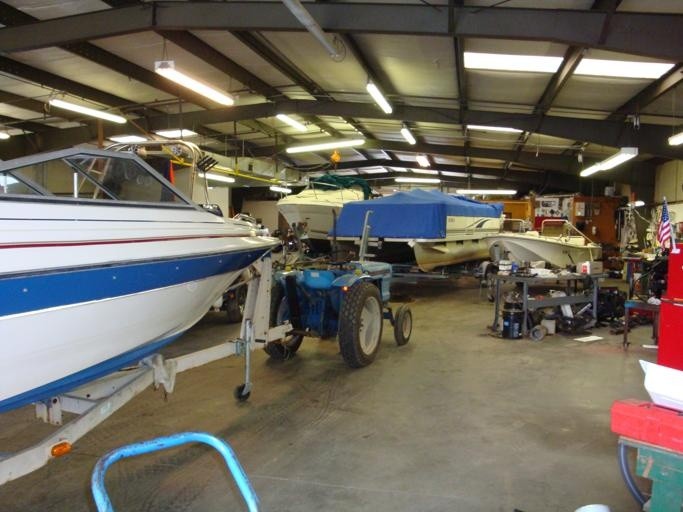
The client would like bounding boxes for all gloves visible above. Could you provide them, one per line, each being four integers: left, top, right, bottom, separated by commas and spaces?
496, 216, 603, 270
0, 145, 279, 412
274, 171, 528, 274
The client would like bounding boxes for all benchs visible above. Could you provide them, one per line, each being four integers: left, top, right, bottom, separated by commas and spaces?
657, 202, 671, 251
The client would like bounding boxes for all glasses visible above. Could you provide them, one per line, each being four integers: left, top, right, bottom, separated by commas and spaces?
49, 89, 127, 124
400, 123, 416, 145
365, 73, 394, 114
153, 36, 236, 106
285, 134, 366, 155
577, 115, 639, 178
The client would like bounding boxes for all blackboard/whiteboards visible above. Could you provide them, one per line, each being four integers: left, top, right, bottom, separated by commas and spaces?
262, 208, 413, 368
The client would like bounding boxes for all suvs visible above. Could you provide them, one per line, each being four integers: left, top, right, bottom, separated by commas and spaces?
503, 310, 524, 338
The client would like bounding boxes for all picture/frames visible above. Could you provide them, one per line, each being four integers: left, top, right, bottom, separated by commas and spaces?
488, 271, 609, 339
